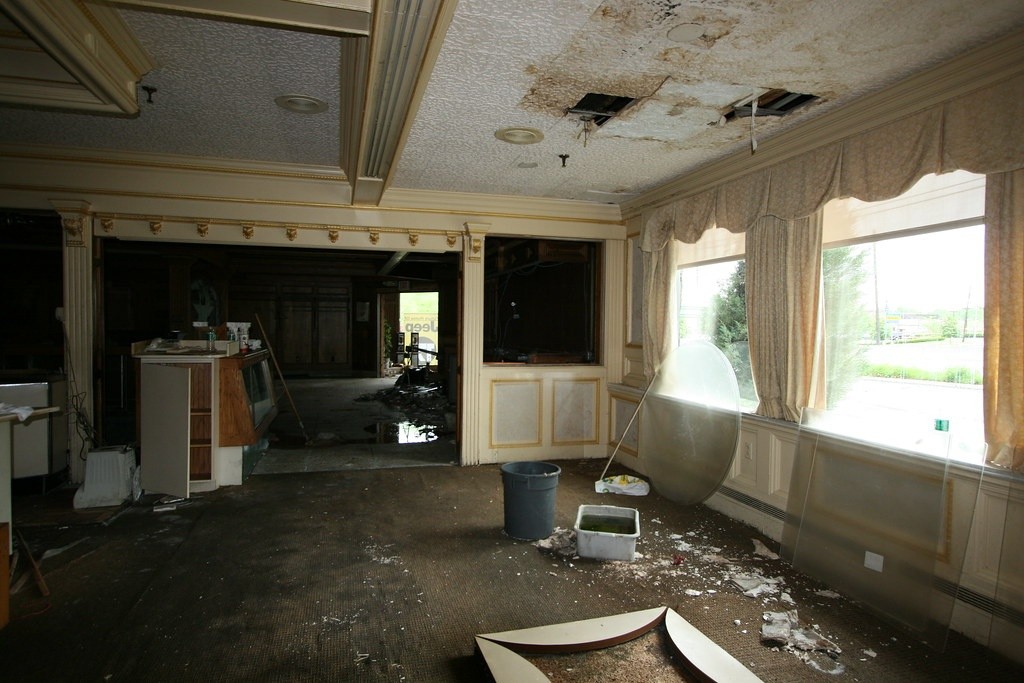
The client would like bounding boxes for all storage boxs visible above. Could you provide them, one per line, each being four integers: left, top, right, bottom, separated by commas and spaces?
574, 504, 641, 560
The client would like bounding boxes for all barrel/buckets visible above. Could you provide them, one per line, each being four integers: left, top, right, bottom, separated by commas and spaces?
500, 461, 561, 538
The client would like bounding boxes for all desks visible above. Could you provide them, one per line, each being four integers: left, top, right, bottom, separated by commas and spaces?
1, 406, 61, 554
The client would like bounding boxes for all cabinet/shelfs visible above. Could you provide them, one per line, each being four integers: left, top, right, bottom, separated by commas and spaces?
226, 273, 353, 378
130, 338, 281, 501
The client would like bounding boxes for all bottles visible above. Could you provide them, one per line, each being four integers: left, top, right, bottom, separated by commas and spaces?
240, 330, 248, 353
228, 330, 235, 340
212, 329, 217, 340
206, 327, 214, 351
